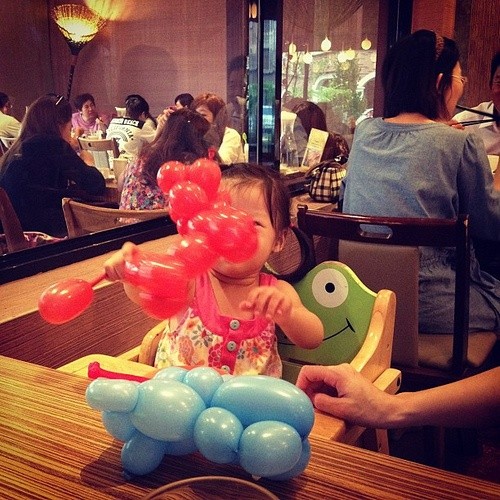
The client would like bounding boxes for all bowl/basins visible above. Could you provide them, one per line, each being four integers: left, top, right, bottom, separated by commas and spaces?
88, 136, 99, 140
487, 154, 499, 173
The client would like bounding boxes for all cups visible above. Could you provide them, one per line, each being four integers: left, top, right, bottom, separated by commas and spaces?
141, 474, 279, 500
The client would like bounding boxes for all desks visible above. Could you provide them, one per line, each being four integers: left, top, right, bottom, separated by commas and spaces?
279, 163, 313, 185
0, 355, 500, 500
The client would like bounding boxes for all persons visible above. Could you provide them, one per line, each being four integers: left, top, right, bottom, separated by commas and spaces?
295, 363, 500, 429
105, 162, 324, 383
0, 89, 244, 240
452, 50, 500, 155
340, 29, 500, 334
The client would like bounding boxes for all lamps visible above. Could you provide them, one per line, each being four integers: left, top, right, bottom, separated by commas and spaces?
50, 4, 107, 102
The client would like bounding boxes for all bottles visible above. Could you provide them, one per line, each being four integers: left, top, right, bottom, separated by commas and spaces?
71, 128, 76, 139
281, 120, 299, 171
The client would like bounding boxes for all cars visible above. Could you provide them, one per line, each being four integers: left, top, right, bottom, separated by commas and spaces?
262, 105, 273, 131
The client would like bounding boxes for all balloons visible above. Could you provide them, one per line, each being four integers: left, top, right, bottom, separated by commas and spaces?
40, 157, 259, 330
86, 367, 313, 482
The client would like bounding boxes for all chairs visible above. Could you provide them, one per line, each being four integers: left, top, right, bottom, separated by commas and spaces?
57, 261, 401, 453
297, 204, 497, 470
0, 140, 169, 255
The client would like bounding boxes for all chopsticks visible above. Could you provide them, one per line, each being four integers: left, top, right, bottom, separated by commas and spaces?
456, 104, 500, 119
459, 119, 497, 127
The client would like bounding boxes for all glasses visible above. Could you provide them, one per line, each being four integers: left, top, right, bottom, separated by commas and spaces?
46, 93, 64, 106
452, 74, 467, 86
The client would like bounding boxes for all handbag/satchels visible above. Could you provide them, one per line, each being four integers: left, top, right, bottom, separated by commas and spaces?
304, 161, 347, 203
